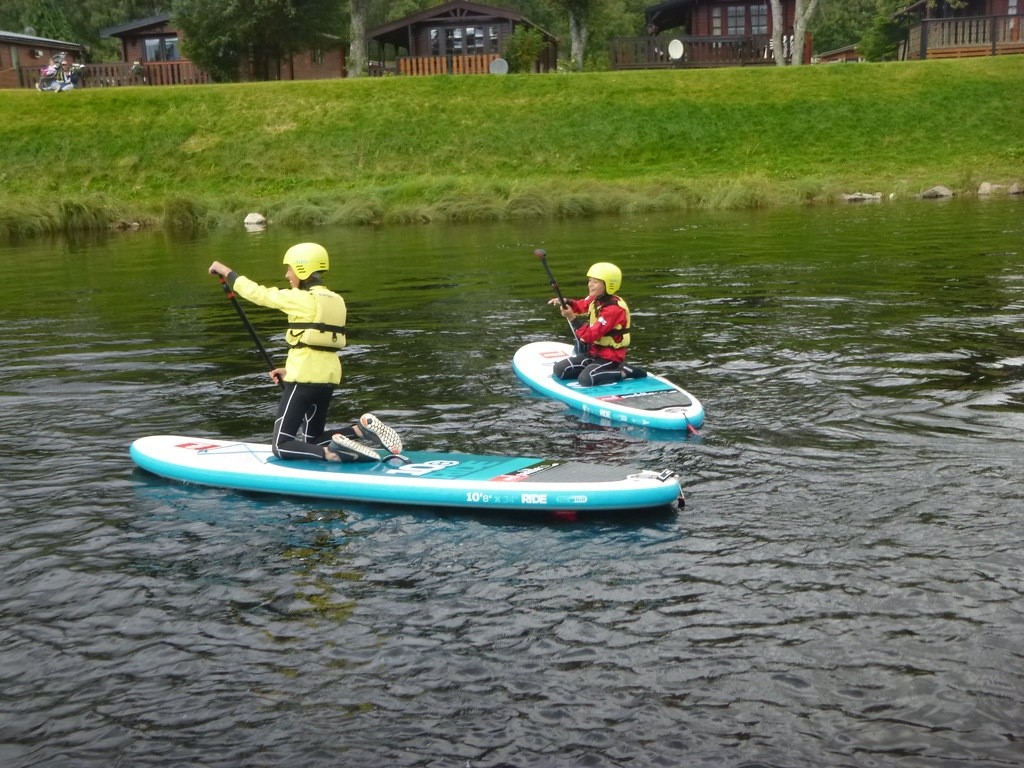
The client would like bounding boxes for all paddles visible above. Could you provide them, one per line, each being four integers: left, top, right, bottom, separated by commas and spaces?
533, 248, 580, 343
211, 270, 287, 392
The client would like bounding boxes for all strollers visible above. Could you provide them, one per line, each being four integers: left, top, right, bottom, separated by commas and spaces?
39, 51, 69, 89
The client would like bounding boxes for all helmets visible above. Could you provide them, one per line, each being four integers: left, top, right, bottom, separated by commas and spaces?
586, 262, 623, 295
283, 243, 329, 280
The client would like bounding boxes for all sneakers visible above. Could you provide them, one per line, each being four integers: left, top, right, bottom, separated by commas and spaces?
327, 434, 381, 463
358, 413, 402, 454
618, 362, 647, 378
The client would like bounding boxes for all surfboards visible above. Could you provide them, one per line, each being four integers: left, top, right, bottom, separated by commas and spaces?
510, 340, 706, 436
128, 432, 686, 514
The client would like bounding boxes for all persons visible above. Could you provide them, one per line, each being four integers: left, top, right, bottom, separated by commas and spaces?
547, 262, 646, 386
208, 243, 403, 463
35, 60, 73, 93
